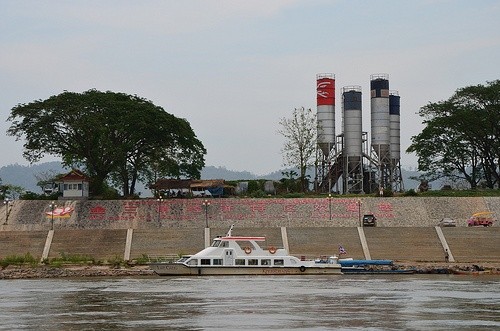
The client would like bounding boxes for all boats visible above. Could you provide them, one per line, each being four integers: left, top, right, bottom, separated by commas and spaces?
449, 268, 492, 274
338, 259, 415, 274
146, 223, 346, 275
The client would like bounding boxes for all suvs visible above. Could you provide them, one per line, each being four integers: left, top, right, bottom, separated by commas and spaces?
362, 214, 378, 226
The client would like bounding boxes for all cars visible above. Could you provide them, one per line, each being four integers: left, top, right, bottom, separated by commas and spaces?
437, 218, 456, 227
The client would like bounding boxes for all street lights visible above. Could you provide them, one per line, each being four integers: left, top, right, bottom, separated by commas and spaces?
326, 194, 333, 221
157, 195, 164, 224
355, 197, 363, 227
48, 201, 57, 230
202, 199, 211, 227
3, 197, 10, 224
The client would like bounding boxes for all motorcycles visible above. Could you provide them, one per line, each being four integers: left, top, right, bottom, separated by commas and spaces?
466, 211, 496, 226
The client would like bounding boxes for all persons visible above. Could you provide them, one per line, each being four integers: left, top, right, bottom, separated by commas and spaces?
379, 186, 383, 197
445, 249, 449, 263
172, 190, 180, 196
340, 246, 346, 254
